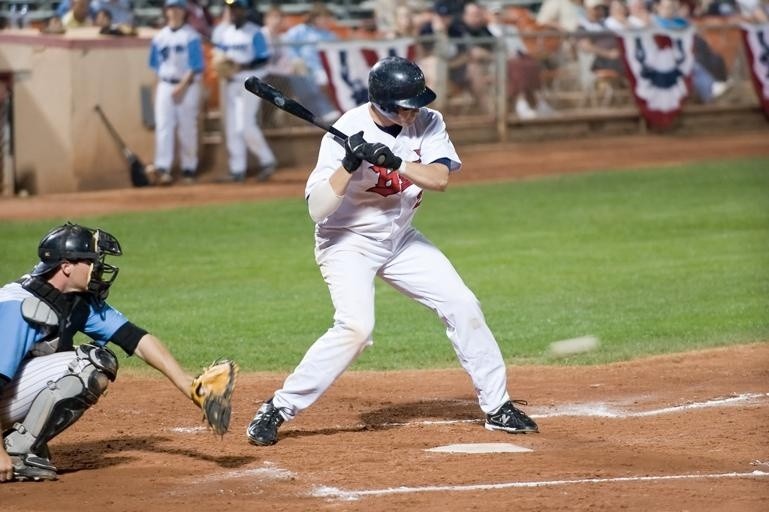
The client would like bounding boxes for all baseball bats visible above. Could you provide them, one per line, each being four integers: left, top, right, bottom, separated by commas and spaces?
243, 75, 386, 164
94, 105, 148, 187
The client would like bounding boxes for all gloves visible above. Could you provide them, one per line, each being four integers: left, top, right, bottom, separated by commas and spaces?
341, 130, 367, 173
355, 142, 403, 171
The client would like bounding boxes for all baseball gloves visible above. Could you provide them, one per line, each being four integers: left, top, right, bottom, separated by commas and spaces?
192, 357, 237, 437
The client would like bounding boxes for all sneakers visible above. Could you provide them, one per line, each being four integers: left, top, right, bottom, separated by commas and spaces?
247, 395, 284, 446
485, 401, 539, 433
8, 454, 56, 481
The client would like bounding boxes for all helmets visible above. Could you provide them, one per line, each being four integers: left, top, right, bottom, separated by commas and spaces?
368, 57, 436, 113
31, 222, 122, 309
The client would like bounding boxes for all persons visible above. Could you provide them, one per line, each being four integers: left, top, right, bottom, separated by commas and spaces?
147, 0, 205, 183
209, 0, 279, 183
247, 57, 538, 446
1, 224, 232, 481
395, 0, 768, 120
50, 0, 368, 123
